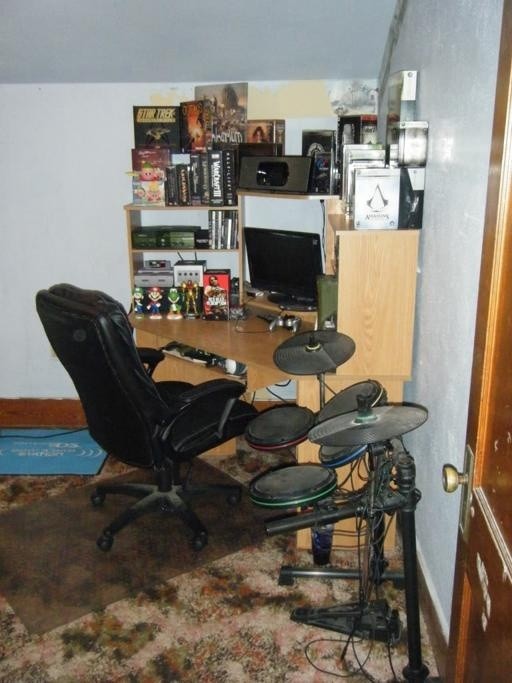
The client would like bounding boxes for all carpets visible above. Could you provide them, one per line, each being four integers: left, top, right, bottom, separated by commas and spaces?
0, 456, 285, 633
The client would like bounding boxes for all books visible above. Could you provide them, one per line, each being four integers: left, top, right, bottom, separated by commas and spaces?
207, 209, 239, 250
165, 148, 237, 207
340, 144, 401, 229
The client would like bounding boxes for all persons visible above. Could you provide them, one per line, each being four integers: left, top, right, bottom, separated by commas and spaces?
204, 275, 228, 318
130, 279, 203, 320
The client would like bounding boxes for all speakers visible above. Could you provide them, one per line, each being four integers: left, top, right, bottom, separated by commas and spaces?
303, 130, 335, 157
238, 155, 314, 194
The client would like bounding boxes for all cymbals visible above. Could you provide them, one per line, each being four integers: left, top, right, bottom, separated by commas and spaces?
272, 331, 355, 375
308, 406, 428, 447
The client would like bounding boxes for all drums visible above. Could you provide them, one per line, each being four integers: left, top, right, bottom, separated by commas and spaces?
243, 405, 315, 451
313, 380, 387, 428
247, 463, 337, 506
319, 446, 370, 469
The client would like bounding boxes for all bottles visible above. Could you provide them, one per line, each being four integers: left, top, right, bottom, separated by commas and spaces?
207, 210, 239, 251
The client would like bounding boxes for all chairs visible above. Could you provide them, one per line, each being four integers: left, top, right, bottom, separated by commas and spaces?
33, 282, 260, 554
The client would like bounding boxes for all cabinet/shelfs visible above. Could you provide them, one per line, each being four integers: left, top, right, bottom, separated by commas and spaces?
124, 190, 420, 552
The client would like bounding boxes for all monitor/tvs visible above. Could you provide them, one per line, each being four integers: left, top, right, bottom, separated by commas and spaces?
245, 227, 323, 310
